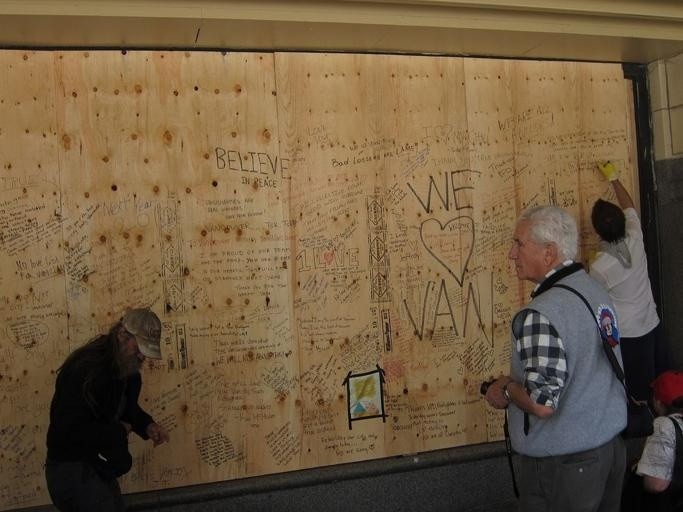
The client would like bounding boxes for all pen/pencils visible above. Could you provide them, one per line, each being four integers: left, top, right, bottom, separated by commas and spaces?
603, 161, 610, 168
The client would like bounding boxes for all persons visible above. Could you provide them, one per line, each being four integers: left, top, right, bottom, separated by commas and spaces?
620, 370, 682, 512
46, 308, 169, 512
479, 204, 628, 511
587, 157, 660, 413
601, 312, 617, 346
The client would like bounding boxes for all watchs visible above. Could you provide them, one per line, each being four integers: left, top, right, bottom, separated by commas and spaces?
503, 380, 513, 407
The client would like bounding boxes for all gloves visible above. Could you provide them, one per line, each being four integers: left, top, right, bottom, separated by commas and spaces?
597, 157, 620, 183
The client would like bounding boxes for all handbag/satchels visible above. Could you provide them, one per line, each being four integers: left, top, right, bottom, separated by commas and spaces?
621, 400, 655, 438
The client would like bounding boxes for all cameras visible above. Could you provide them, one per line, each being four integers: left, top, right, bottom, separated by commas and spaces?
479, 379, 509, 409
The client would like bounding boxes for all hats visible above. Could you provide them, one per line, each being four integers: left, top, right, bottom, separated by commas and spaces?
649, 369, 683, 409
121, 306, 163, 360
